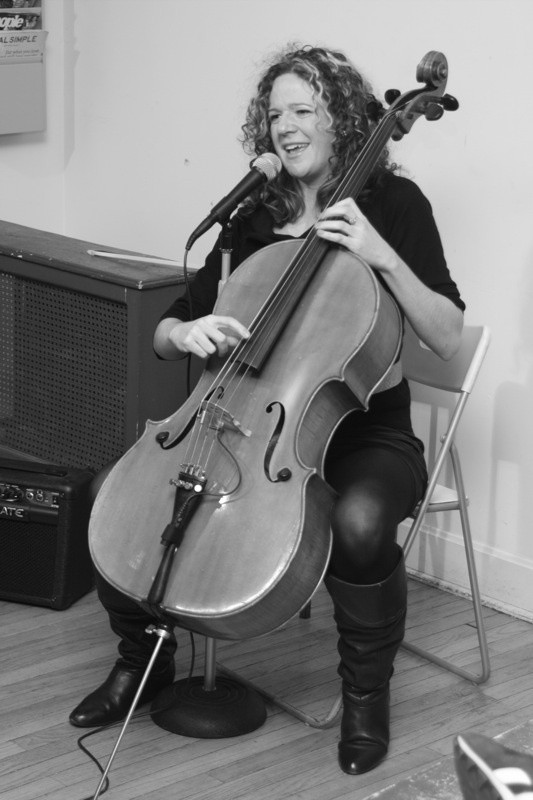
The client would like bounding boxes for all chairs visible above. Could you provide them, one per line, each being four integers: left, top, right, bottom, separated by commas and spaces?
213, 325, 491, 730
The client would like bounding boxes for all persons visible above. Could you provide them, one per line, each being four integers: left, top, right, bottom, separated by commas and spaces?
68, 44, 468, 774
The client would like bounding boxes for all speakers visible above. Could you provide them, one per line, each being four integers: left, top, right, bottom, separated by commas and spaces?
0, 221, 204, 612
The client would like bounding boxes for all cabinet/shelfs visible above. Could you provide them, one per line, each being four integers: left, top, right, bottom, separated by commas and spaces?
0, 0, 45, 136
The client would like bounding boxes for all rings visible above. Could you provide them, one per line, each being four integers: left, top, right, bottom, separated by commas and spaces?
349, 216, 358, 224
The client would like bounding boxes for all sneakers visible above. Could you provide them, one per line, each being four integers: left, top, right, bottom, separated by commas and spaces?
454, 732, 533, 800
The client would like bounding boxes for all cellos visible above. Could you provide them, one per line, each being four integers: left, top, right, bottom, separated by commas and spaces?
89, 47, 459, 800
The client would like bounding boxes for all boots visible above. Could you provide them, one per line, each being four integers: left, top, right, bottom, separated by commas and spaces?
69, 569, 176, 728
322, 544, 408, 776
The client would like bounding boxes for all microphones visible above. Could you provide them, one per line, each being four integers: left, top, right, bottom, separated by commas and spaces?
185, 152, 281, 251
